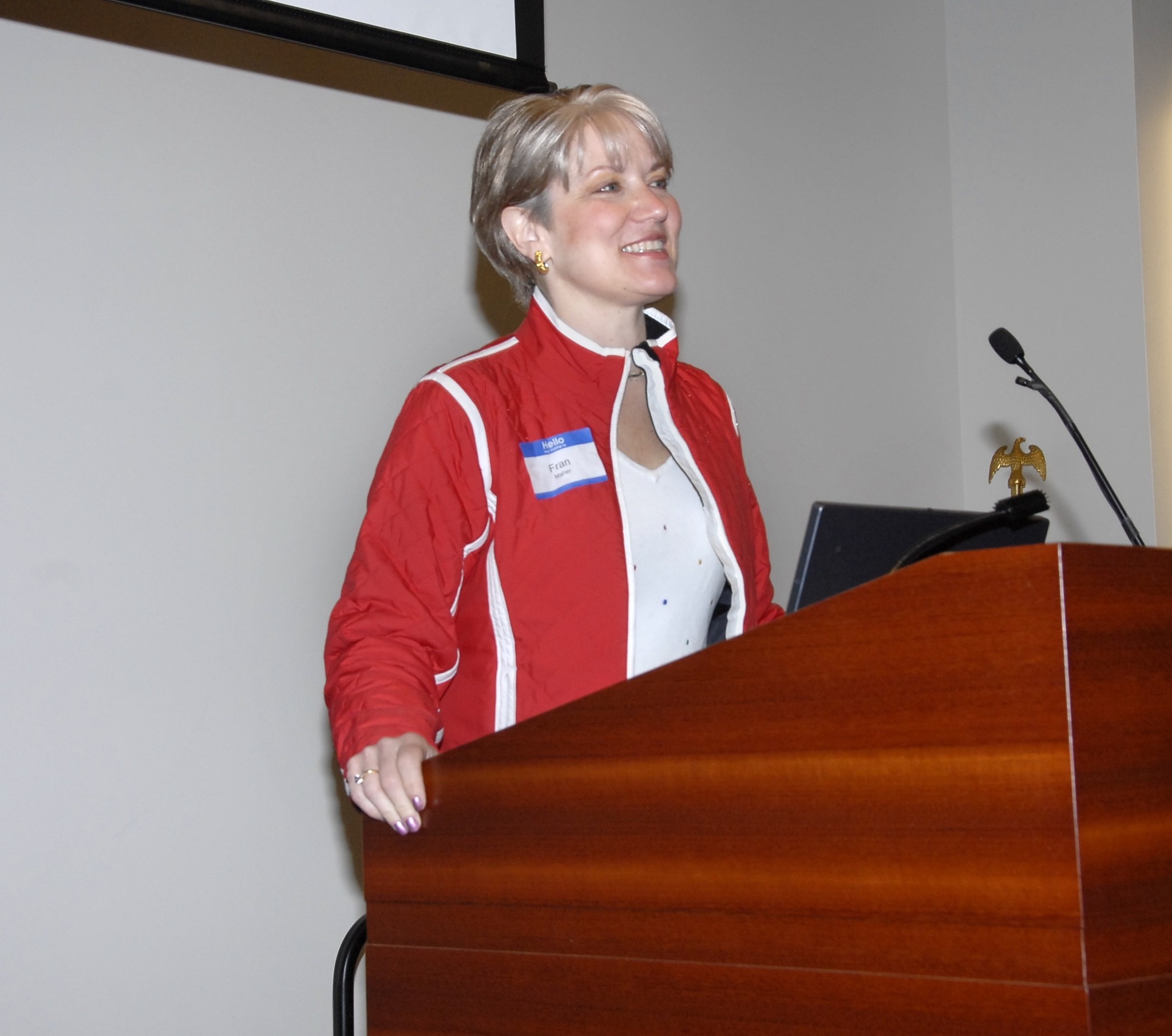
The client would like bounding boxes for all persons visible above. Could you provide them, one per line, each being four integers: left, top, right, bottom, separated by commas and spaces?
323, 85, 784, 835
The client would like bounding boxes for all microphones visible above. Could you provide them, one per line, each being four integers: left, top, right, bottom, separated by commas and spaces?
990, 327, 1142, 546
896, 490, 1050, 572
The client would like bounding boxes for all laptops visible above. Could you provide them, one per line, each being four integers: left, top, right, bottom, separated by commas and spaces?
788, 502, 1048, 618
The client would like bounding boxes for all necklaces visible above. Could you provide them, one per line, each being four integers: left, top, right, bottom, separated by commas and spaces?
627, 370, 645, 380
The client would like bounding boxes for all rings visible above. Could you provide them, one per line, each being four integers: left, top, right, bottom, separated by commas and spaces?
353, 768, 379, 785
